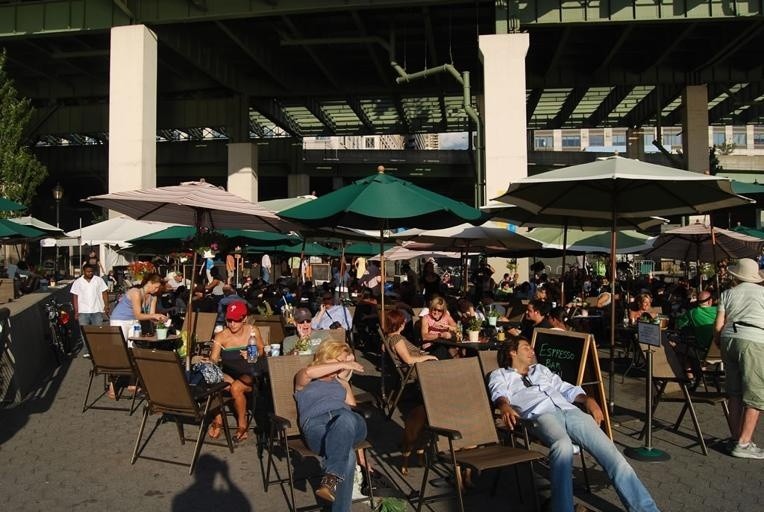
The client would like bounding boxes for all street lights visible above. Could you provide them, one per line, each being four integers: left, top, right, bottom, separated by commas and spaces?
52, 180, 64, 281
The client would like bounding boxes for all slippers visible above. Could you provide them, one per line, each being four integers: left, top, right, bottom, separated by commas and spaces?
232, 427, 250, 442
208, 421, 223, 441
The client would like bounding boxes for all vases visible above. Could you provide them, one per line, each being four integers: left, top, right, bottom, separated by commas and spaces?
198, 247, 216, 257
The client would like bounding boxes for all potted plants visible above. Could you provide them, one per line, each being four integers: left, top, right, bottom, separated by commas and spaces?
486, 311, 500, 326
467, 320, 480, 342
155, 318, 168, 339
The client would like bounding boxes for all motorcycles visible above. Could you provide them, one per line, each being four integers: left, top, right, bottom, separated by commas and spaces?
45, 300, 74, 353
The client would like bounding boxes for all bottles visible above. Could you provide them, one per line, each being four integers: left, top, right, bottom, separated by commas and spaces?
456, 320, 463, 342
134, 320, 142, 338
248, 331, 259, 363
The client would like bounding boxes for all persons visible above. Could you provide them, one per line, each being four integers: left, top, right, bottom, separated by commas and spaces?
420, 296, 464, 358
294, 340, 369, 511
546, 306, 578, 333
383, 309, 438, 384
199, 301, 264, 441
487, 337, 660, 512
669, 292, 718, 371
0, 256, 57, 299
217, 282, 254, 326
458, 299, 488, 327
232, 250, 339, 318
519, 301, 555, 337
713, 258, 764, 460
469, 264, 634, 349
477, 291, 506, 318
162, 285, 203, 312
69, 263, 112, 358
684, 253, 738, 294
205, 265, 226, 303
106, 273, 162, 399
274, 255, 381, 354
164, 270, 197, 291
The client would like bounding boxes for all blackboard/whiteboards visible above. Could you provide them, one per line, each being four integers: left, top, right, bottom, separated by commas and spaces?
527, 328, 590, 387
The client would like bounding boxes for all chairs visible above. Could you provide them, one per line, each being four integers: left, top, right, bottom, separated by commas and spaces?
251, 314, 286, 348
209, 325, 271, 436
81, 326, 146, 415
129, 349, 234, 474
176, 312, 218, 359
505, 305, 526, 328
636, 332, 734, 456
688, 332, 727, 396
376, 325, 420, 421
412, 307, 429, 340
266, 353, 373, 512
379, 305, 395, 329
415, 357, 544, 512
478, 349, 593, 510
345, 307, 357, 362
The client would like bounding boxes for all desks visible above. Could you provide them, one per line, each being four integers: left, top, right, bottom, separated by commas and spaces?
435, 338, 503, 358
619, 322, 670, 385
129, 334, 181, 351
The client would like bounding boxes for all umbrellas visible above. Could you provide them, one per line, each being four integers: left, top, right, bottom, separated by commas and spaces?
716, 170, 762, 204
527, 228, 669, 333
274, 165, 492, 399
2, 219, 63, 245
127, 226, 305, 245
44, 195, 192, 245
4, 214, 62, 233
82, 179, 307, 243
1, 196, 30, 214
732, 223, 763, 240
250, 196, 319, 217
634, 224, 764, 308
237, 239, 340, 255
320, 224, 427, 274
397, 224, 544, 296
492, 150, 763, 417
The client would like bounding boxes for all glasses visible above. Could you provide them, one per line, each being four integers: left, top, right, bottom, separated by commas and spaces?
227, 318, 244, 323
323, 297, 333, 301
300, 320, 311, 324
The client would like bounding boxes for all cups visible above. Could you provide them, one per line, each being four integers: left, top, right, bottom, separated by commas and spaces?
496, 333, 504, 341
270, 343, 279, 356
163, 319, 171, 327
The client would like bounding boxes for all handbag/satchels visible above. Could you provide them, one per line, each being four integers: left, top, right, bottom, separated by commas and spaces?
325, 309, 341, 329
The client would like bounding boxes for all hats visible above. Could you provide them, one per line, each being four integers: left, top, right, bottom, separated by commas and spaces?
225, 300, 248, 321
725, 257, 764, 284
293, 307, 312, 323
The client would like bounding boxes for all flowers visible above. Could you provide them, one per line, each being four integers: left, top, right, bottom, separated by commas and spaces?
183, 226, 233, 250
128, 260, 155, 276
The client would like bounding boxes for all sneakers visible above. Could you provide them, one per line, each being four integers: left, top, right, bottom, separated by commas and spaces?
315, 471, 339, 503
732, 440, 764, 462
726, 438, 756, 453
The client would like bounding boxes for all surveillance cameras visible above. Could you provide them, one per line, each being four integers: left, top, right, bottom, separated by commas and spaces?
403, 137, 415, 149
